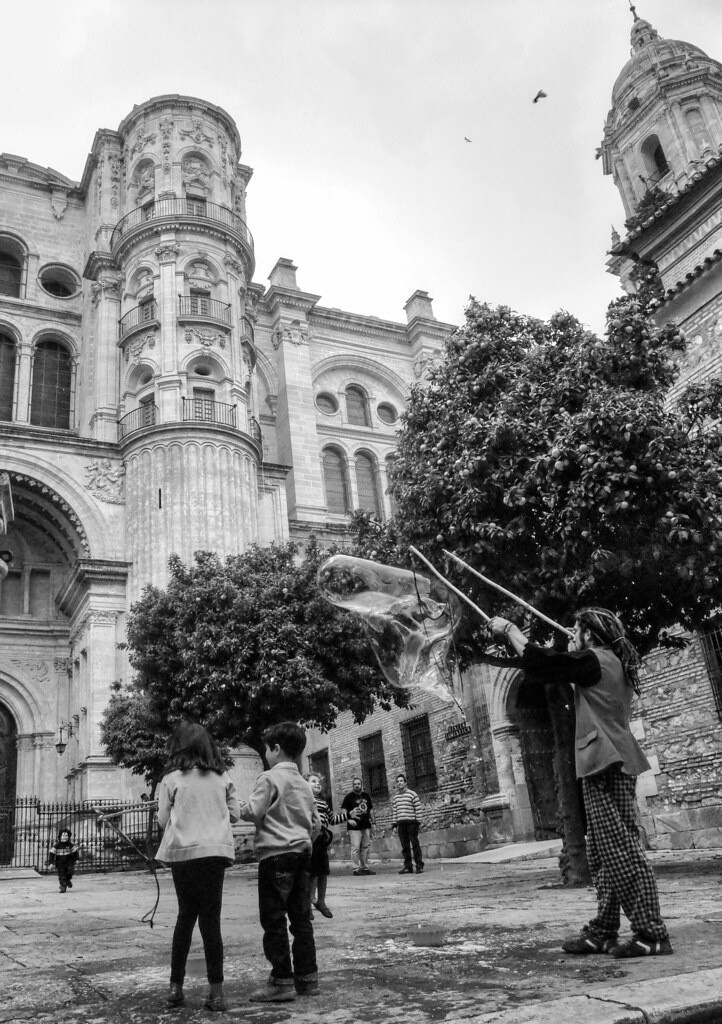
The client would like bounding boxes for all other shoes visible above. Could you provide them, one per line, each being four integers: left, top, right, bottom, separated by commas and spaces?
206, 991, 226, 1011
415, 865, 423, 873
310, 905, 314, 920
296, 980, 320, 996
166, 983, 184, 1006
249, 982, 297, 1002
60, 887, 66, 893
399, 867, 413, 874
67, 881, 72, 888
353, 869, 376, 875
314, 901, 333, 918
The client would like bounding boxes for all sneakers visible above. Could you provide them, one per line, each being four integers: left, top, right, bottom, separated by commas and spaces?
609, 933, 674, 957
562, 925, 620, 954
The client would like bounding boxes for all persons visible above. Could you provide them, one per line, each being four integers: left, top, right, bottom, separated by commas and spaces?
391, 774, 424, 875
487, 607, 673, 957
155, 724, 243, 1012
340, 777, 377, 875
238, 722, 322, 1001
302, 772, 362, 920
48, 828, 79, 893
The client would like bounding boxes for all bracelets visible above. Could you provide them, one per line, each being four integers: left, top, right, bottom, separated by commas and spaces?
372, 822, 377, 824
503, 622, 514, 645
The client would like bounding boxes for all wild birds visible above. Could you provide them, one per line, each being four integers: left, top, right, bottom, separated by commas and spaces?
465, 137, 472, 142
533, 90, 547, 104
